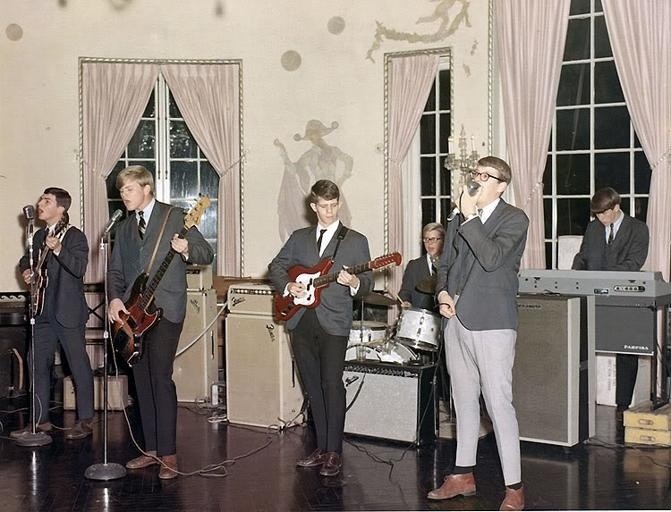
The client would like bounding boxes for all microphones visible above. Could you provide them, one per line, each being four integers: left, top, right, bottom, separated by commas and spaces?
466, 180, 481, 197
23, 205, 34, 220
101, 209, 123, 239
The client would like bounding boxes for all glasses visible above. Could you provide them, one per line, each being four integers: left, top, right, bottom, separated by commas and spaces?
470, 169, 503, 182
423, 236, 441, 242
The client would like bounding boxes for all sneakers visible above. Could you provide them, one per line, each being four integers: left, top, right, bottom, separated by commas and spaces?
616, 405, 628, 422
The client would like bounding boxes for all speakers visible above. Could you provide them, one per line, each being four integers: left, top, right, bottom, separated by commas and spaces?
512, 292, 596, 446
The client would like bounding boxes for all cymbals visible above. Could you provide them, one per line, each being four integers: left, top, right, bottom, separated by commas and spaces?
415, 276, 436, 294
359, 290, 398, 307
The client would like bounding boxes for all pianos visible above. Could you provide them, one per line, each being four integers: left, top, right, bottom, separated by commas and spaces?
518, 269, 670, 306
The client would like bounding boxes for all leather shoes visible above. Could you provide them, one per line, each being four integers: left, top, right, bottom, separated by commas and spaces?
65, 421, 94, 439
427, 472, 476, 499
321, 452, 343, 476
126, 451, 161, 469
10, 421, 51, 439
297, 447, 326, 467
159, 454, 178, 479
499, 483, 525, 511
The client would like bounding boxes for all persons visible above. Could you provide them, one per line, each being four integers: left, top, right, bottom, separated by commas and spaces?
106, 165, 214, 478
10, 188, 94, 440
426, 157, 529, 510
274, 120, 353, 243
267, 179, 370, 476
397, 223, 445, 313
572, 187, 650, 422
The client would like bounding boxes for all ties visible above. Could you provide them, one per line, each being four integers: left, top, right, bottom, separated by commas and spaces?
431, 263, 437, 278
315, 229, 327, 252
43, 228, 49, 242
477, 208, 483, 219
138, 211, 147, 240
608, 224, 614, 243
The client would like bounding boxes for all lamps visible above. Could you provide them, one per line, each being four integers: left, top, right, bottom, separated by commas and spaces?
444, 123, 478, 182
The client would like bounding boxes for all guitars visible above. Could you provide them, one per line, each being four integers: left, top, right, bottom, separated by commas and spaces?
273, 252, 403, 321
29, 211, 69, 316
111, 193, 210, 367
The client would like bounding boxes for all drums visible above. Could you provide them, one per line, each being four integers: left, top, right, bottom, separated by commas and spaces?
345, 341, 419, 363
348, 320, 389, 344
395, 308, 443, 351
